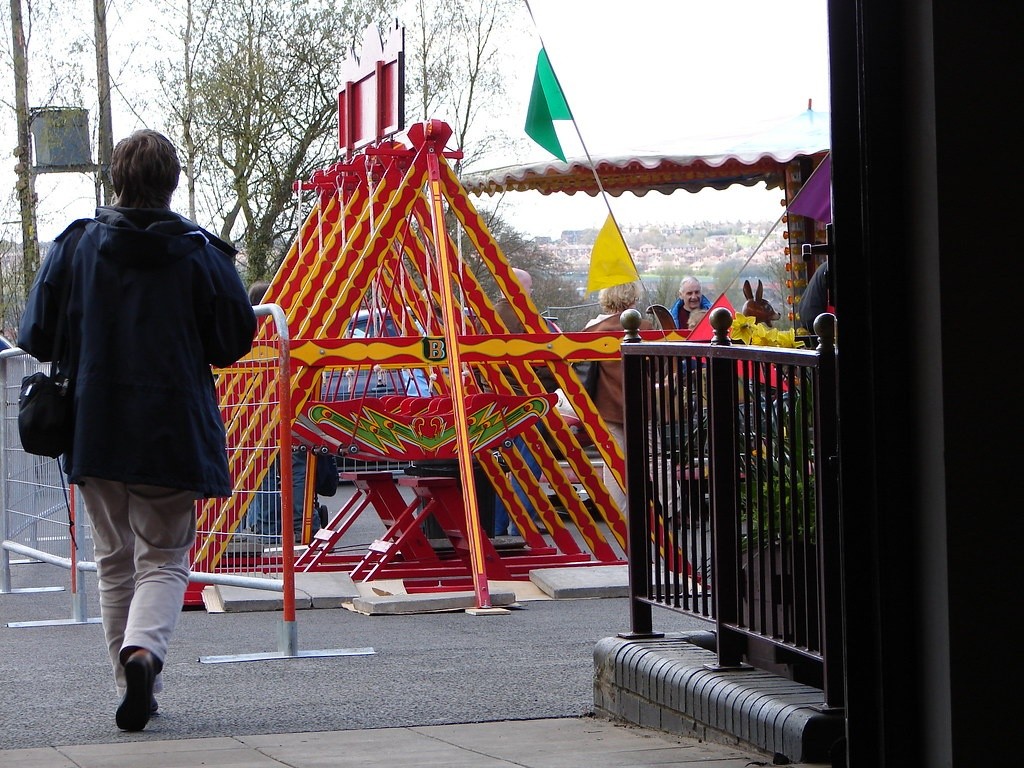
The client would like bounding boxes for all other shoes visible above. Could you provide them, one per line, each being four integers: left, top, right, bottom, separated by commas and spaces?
232, 527, 247, 542
671, 511, 700, 528
512, 526, 549, 536
296, 532, 316, 543
115, 650, 159, 732
259, 533, 279, 544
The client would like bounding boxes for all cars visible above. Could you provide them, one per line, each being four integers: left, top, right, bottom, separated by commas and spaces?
320, 306, 463, 442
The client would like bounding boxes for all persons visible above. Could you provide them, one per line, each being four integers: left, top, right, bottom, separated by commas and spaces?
244, 281, 322, 542
669, 277, 711, 521
578, 281, 686, 535
478, 265, 558, 538
400, 287, 484, 533
16, 130, 256, 734
0, 329, 9, 350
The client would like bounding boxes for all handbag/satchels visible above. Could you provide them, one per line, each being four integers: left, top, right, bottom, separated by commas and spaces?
570, 360, 597, 400
18, 372, 73, 460
